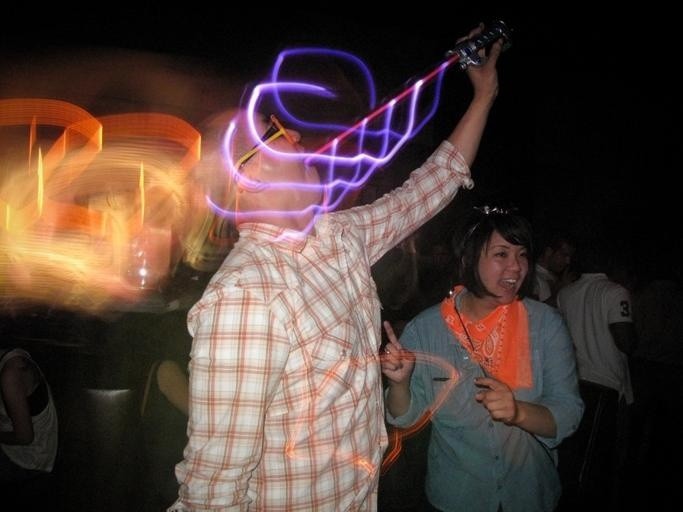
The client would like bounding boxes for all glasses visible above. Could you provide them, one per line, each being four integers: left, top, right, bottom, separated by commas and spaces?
231, 111, 299, 171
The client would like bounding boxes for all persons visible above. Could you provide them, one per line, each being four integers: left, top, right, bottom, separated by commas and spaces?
0, 196, 683, 511
140, 24, 506, 512
